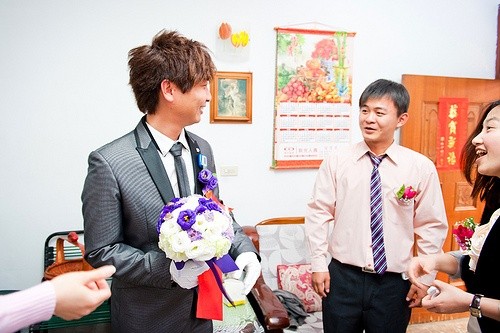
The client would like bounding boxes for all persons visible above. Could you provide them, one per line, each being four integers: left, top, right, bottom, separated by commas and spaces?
407, 100, 500, 333
81, 31, 261, 333
0, 265, 116, 333
304, 79, 449, 333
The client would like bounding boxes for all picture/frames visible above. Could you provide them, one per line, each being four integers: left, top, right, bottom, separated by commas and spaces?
209, 70, 253, 124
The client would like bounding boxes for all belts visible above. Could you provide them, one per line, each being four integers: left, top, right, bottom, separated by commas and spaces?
333, 258, 401, 277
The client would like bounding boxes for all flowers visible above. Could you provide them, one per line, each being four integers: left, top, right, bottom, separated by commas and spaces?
155, 194, 234, 271
452, 215, 477, 250
396, 184, 417, 204
197, 168, 221, 193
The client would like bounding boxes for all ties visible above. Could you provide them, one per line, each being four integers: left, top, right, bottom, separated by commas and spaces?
169, 143, 192, 197
367, 152, 389, 276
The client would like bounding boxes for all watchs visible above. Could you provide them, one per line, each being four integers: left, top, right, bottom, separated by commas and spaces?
468, 294, 484, 318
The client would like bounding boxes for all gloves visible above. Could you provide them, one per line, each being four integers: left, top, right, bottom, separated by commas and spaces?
231, 251, 262, 295
170, 260, 210, 289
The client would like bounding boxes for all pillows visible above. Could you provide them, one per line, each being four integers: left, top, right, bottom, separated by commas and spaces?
276, 263, 322, 312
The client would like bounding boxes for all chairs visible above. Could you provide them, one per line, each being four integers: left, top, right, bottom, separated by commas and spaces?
241, 216, 324, 333
30, 229, 113, 333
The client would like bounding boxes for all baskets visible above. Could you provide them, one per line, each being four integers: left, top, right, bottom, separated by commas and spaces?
43, 232, 95, 281
